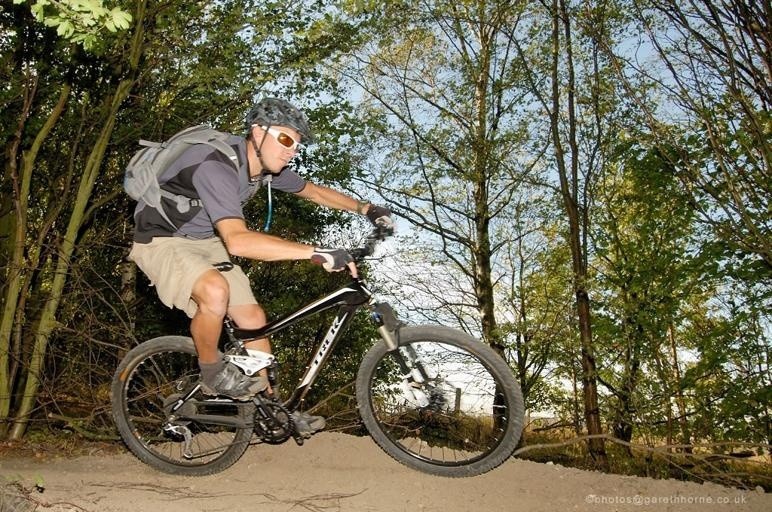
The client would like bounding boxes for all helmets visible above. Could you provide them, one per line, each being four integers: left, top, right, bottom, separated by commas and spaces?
246, 97, 313, 138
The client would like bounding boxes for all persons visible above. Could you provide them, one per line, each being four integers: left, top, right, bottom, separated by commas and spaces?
128, 96, 392, 435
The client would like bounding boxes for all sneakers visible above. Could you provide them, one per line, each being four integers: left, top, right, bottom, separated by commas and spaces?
278, 412, 325, 434
202, 363, 268, 398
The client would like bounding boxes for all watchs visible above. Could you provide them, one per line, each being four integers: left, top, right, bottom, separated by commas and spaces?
357, 198, 372, 215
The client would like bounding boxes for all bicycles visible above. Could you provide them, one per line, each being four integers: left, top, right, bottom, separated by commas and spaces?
110, 220, 525, 479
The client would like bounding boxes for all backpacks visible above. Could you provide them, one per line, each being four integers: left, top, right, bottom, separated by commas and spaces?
122, 123, 241, 231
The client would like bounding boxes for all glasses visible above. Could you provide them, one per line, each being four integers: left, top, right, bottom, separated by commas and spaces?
252, 123, 307, 154
